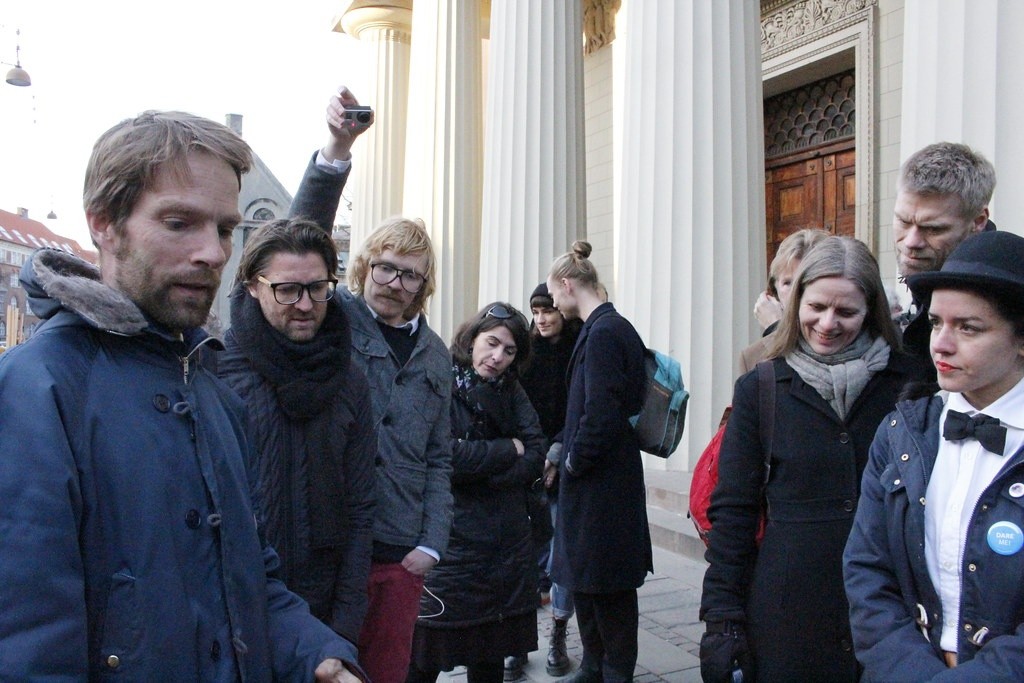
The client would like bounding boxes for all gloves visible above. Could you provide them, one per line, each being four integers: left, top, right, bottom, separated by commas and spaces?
700, 606, 749, 683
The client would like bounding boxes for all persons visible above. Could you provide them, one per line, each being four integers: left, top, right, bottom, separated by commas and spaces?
410, 256, 653, 683
699, 139, 1024, 683
0, 110, 368, 683
286, 93, 454, 683
218, 216, 381, 646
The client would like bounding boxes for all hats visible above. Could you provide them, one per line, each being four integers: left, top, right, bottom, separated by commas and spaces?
904, 232, 1024, 298
530, 282, 552, 300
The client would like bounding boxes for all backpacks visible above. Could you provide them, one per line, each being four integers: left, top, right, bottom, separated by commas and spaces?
630, 345, 690, 458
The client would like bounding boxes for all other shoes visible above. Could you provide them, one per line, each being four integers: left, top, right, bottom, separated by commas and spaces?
538, 587, 552, 607
554, 669, 599, 682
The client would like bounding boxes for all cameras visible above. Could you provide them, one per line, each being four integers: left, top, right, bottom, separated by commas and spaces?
343, 106, 372, 127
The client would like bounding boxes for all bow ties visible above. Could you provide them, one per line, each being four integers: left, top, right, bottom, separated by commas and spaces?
942, 409, 1008, 456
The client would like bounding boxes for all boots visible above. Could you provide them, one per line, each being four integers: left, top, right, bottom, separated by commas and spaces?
501, 653, 528, 679
546, 614, 570, 677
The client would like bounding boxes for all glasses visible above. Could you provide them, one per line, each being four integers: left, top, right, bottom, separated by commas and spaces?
254, 271, 338, 305
486, 305, 530, 334
370, 260, 427, 294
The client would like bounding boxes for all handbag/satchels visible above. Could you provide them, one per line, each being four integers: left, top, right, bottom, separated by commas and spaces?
688, 359, 777, 550
